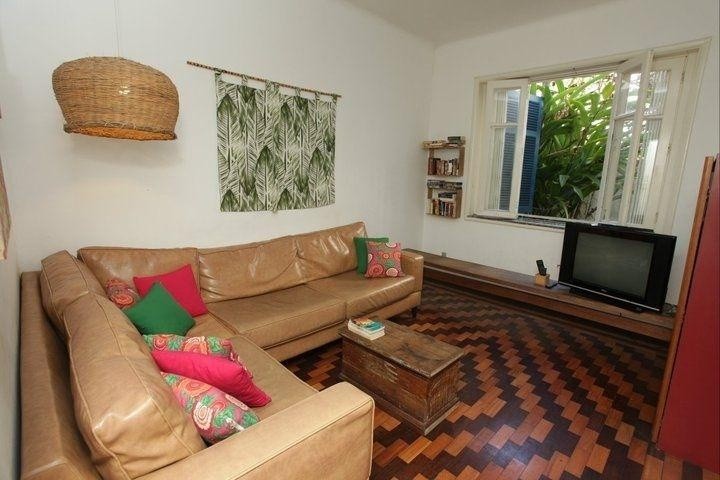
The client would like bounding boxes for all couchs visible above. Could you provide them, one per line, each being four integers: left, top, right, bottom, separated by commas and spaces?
19, 251, 424, 479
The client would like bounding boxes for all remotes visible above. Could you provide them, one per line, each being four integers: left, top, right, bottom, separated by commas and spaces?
536, 260, 546, 276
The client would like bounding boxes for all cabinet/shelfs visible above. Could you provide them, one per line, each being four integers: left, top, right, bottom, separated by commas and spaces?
652, 153, 720, 474
427, 146, 464, 218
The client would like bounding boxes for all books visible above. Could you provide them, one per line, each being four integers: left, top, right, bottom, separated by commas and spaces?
347, 316, 385, 340
422, 137, 465, 217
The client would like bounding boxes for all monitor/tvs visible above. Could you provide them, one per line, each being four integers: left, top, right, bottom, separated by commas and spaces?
558, 222, 677, 313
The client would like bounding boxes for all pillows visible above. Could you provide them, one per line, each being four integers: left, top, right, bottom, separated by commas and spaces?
161, 372, 261, 446
77, 246, 200, 304
142, 333, 252, 379
39, 250, 110, 340
134, 264, 208, 317
61, 291, 208, 480
105, 279, 140, 309
353, 237, 389, 276
151, 350, 272, 408
122, 282, 195, 336
198, 221, 368, 304
364, 240, 405, 278
